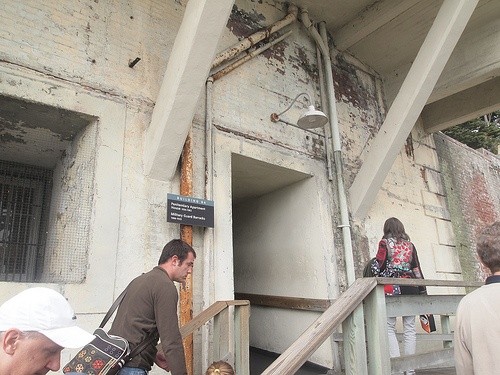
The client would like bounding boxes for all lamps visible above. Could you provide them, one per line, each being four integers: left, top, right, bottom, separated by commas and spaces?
271, 92, 329, 131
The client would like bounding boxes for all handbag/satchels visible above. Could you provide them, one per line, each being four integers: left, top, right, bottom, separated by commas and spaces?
62, 328, 131, 375
420, 314, 436, 333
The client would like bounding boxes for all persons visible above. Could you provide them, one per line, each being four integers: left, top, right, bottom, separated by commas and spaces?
106, 239, 196, 375
373, 217, 428, 375
0, 286, 97, 375
207, 362, 235, 375
453, 221, 500, 375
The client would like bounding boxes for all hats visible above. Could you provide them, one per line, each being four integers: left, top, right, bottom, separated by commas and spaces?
0, 287, 96, 348
